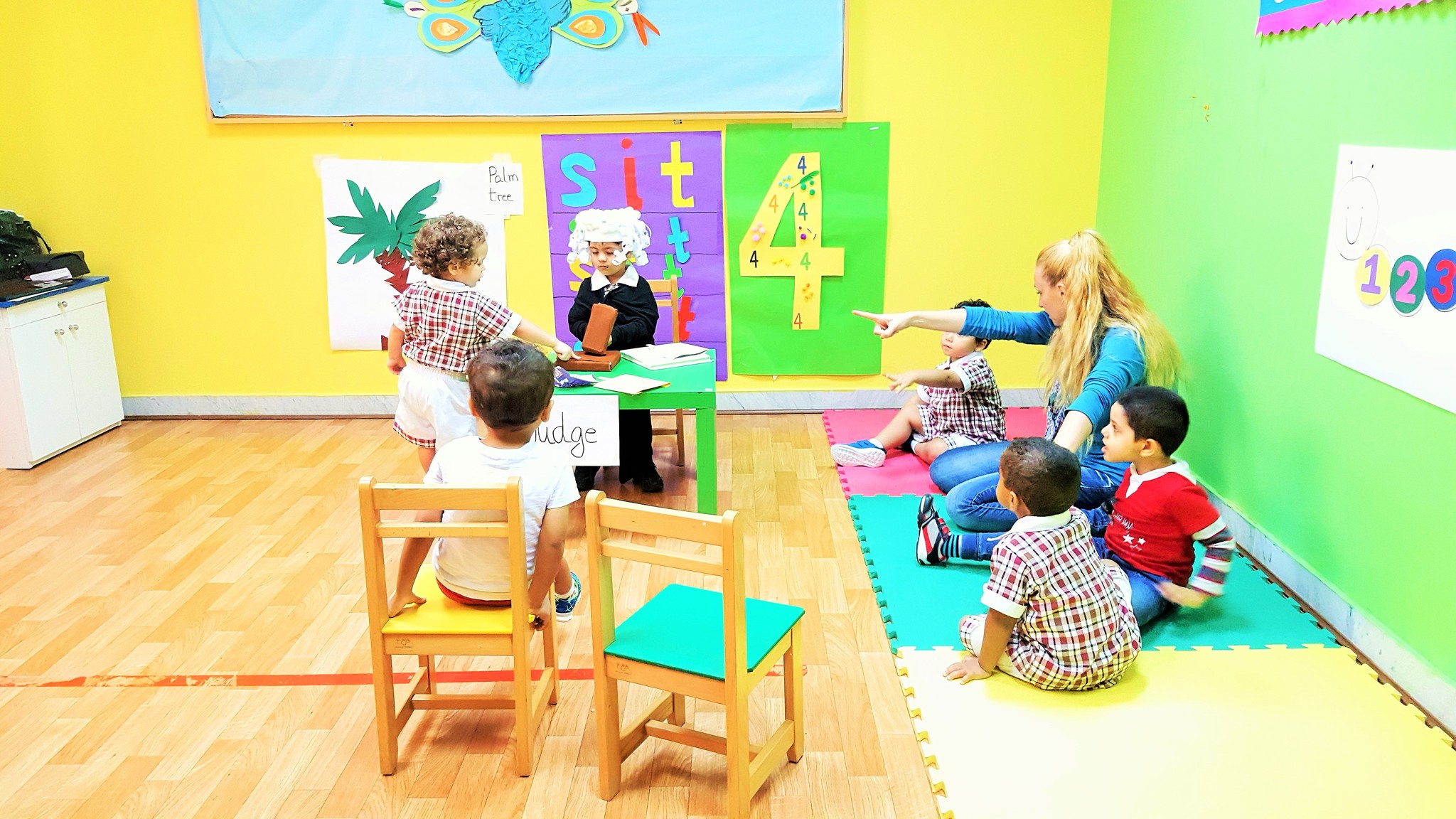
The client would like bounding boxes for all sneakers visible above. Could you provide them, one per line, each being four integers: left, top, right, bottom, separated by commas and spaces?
830, 438, 887, 468
554, 571, 582, 623
916, 493, 953, 568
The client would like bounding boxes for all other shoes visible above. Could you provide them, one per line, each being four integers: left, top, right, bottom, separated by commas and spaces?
574, 466, 597, 492
632, 462, 664, 493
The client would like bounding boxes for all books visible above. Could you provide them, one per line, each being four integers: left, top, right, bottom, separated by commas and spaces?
620, 341, 711, 369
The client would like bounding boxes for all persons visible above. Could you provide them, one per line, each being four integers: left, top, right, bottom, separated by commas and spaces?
566, 206, 667, 496
828, 296, 1008, 467
849, 228, 1183, 532
386, 211, 580, 478
940, 436, 1144, 692
915, 385, 1237, 629
384, 336, 581, 630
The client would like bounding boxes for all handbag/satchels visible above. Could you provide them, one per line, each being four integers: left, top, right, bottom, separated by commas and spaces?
0, 210, 51, 283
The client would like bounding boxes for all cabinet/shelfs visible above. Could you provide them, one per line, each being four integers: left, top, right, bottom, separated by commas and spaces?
1, 274, 125, 471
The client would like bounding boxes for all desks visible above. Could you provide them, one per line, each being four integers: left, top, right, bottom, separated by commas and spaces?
542, 348, 721, 512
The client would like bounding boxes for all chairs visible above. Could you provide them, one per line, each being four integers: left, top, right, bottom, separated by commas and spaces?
354, 471, 560, 776
573, 275, 690, 470
576, 487, 809, 819
